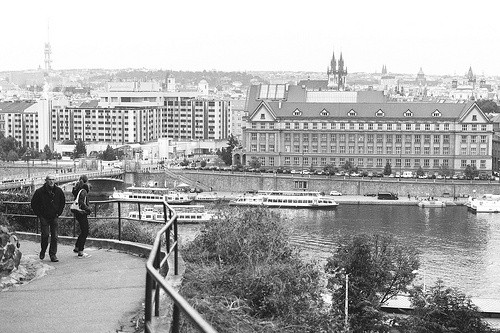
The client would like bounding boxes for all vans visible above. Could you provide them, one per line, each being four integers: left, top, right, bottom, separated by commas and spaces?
377, 192, 399, 200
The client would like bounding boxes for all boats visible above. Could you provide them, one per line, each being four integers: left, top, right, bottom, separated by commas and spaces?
229, 190, 340, 208
108, 187, 193, 204
129, 205, 231, 225
195, 191, 220, 202
418, 198, 445, 208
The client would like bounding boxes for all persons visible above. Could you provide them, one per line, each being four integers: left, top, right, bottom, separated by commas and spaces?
70, 175, 92, 259
31, 174, 65, 262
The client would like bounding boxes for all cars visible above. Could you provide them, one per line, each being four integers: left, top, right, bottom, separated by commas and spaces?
108, 162, 122, 168
364, 191, 376, 196
180, 165, 500, 181
329, 190, 343, 196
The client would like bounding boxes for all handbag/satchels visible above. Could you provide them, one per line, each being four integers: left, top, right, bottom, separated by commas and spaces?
70, 189, 90, 215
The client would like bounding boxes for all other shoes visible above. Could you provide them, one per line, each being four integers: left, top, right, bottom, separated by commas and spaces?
72, 250, 87, 256
50, 255, 58, 262
78, 253, 92, 259
40, 252, 45, 259
